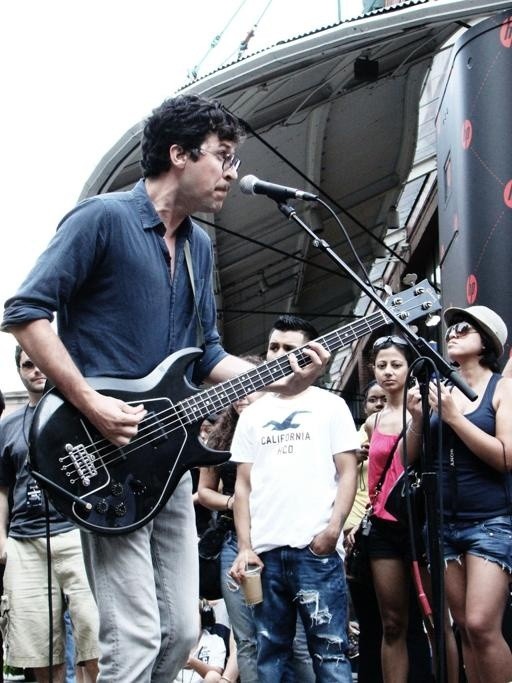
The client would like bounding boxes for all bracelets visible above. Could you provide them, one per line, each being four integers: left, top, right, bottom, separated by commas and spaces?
407, 423, 422, 440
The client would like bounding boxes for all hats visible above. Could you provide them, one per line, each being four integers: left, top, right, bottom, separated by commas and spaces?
442, 304, 509, 359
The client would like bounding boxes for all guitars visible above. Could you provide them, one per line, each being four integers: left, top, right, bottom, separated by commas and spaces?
27, 272, 440, 533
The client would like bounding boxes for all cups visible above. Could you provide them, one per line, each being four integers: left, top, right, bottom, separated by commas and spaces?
237, 560, 265, 606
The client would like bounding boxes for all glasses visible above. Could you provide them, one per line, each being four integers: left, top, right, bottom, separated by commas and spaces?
197, 147, 241, 172
443, 322, 478, 343
373, 334, 409, 347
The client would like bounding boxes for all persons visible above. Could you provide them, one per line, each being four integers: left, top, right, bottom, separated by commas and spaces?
0, 341, 109, 681
1, 91, 336, 681
396, 303, 512, 680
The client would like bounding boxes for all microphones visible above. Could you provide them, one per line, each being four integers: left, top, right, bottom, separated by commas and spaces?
239, 174, 318, 203
